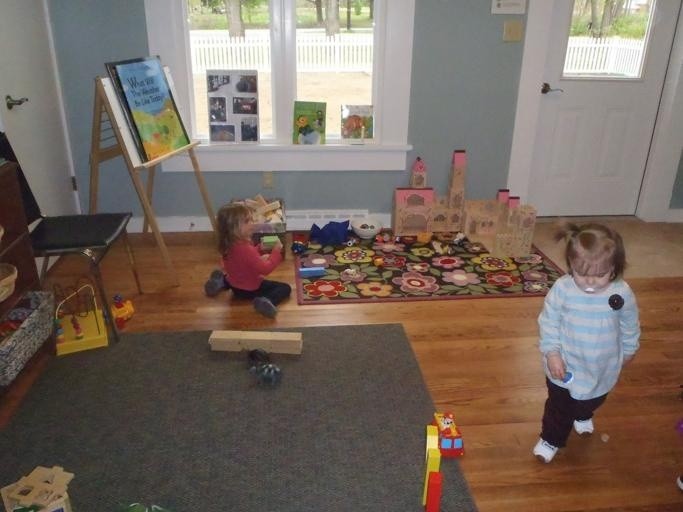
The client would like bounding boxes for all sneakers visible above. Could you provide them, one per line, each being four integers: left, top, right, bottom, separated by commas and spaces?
254, 297, 277, 319
206, 270, 224, 297
574, 418, 594, 436
534, 439, 558, 463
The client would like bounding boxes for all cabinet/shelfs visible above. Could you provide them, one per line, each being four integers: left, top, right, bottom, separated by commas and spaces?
1, 162, 44, 321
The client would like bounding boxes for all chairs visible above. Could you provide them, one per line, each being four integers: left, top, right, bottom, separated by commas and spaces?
1, 131, 142, 343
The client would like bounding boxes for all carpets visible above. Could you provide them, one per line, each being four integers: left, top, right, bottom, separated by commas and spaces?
292, 229, 566, 305
1, 323, 480, 512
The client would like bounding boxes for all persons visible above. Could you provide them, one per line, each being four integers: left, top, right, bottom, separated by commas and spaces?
205, 203, 291, 318
533, 219, 642, 463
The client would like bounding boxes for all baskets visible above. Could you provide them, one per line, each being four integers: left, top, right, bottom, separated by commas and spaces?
0, 263, 18, 302
